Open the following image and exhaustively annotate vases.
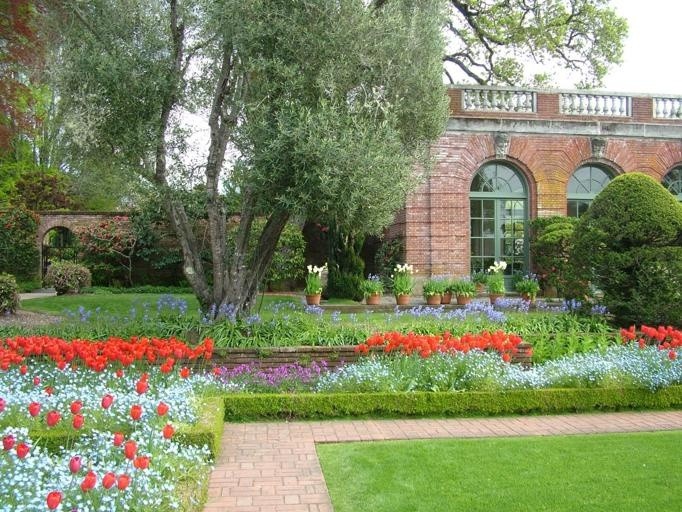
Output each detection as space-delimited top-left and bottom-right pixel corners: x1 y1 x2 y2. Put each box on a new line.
489 293 504 304
305 294 321 306
365 293 381 305
395 294 411 305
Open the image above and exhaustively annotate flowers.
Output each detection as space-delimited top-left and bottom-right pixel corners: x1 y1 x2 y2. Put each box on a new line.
303 264 323 296
389 263 420 299
487 261 508 293
361 272 385 298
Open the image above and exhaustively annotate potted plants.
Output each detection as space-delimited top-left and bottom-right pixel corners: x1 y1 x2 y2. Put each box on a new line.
517 279 540 301
422 271 489 305
